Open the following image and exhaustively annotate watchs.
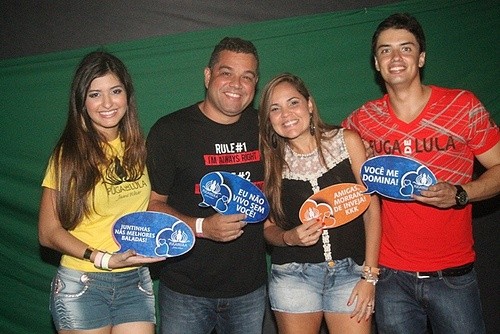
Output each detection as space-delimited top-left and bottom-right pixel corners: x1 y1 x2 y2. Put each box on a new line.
453 184 469 209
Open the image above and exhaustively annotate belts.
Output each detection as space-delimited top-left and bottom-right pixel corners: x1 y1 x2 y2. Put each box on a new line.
407 261 475 279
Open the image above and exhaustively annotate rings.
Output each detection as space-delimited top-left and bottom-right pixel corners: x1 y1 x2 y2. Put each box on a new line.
367 305 372 307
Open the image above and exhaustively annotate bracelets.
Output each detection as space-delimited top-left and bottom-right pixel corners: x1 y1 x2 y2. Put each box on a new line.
195 217 204 238
360 265 380 286
101 252 112 271
83 247 98 263
282 230 291 246
94 251 105 269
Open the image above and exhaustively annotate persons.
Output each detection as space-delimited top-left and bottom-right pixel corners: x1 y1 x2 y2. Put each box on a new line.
144 35 269 334
38 50 166 334
257 70 383 334
341 12 500 334
